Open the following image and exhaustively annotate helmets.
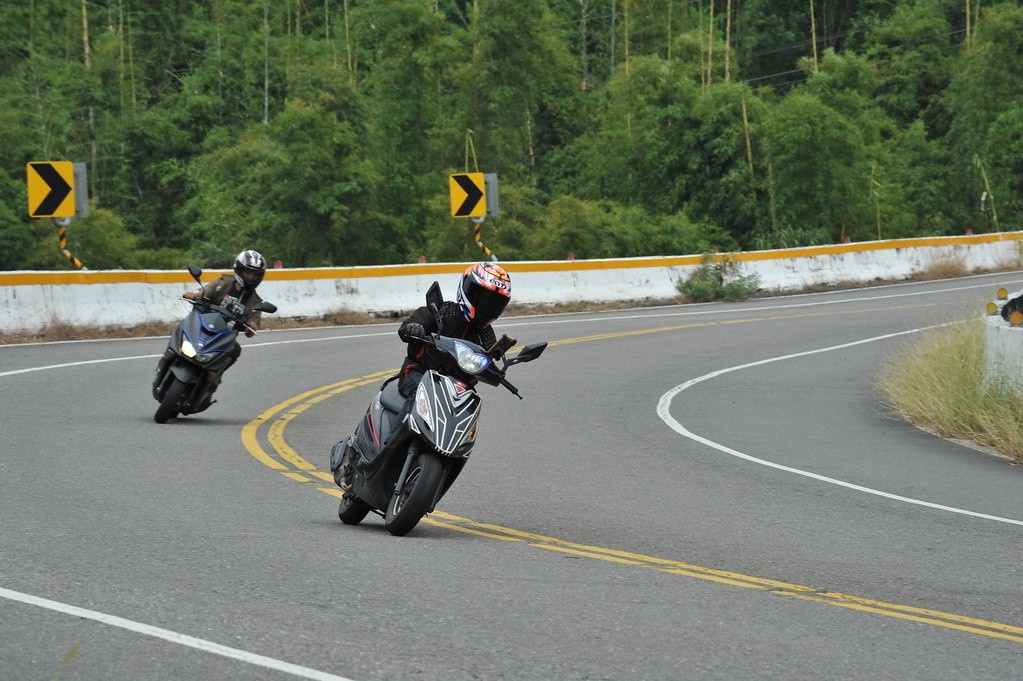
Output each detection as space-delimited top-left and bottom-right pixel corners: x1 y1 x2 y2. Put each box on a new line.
234 249 267 290
457 262 512 329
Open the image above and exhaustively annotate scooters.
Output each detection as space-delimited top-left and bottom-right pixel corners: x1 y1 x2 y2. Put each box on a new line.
151 264 278 424
328 279 549 537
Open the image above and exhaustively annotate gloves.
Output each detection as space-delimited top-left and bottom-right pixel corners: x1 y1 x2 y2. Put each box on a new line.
245 321 257 338
404 322 426 341
184 291 201 300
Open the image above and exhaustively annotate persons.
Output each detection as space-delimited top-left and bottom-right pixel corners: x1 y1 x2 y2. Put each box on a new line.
156 250 265 394
384 261 510 462
